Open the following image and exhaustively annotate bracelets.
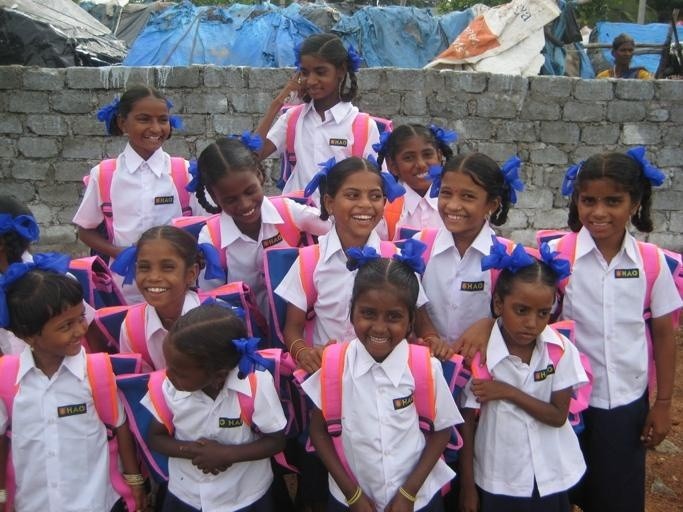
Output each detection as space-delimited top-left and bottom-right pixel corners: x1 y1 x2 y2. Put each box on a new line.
288 337 305 356
122 472 146 486
422 332 441 340
655 397 673 402
294 345 313 365
348 487 361 505
398 486 416 502
342 484 359 504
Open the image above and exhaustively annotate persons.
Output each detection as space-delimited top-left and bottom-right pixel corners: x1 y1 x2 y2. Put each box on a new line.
410 152 518 511
536 148 682 511
0 191 94 355
456 230 591 512
595 34 653 79
0 266 150 512
185 133 333 334
371 122 455 239
295 236 465 510
68 85 219 305
137 297 289 511
275 154 455 378
247 33 389 204
109 222 239 374
